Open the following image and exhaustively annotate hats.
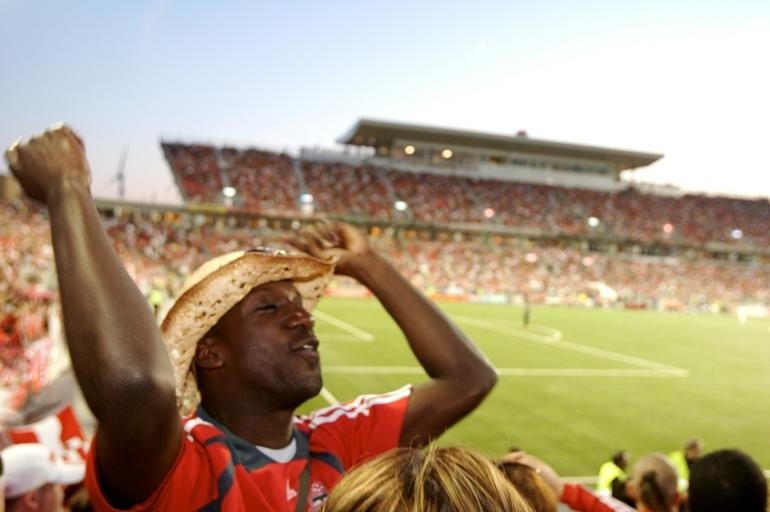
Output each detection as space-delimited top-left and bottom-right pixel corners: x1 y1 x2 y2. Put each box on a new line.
157 246 347 415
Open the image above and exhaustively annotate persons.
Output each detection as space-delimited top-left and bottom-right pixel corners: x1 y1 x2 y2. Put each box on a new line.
0 182 162 512
8 126 499 512
319 438 769 512
163 143 769 317
